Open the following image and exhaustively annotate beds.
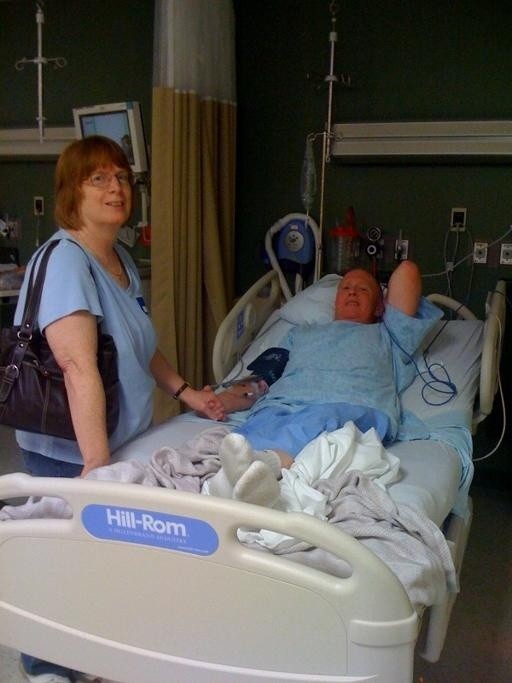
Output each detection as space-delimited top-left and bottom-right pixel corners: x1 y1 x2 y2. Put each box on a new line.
1 275 507 683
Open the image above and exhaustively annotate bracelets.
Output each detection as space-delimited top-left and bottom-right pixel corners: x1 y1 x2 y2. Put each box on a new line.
173 381 193 400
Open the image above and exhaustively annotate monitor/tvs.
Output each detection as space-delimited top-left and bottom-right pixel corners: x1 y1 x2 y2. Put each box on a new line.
72 101 149 173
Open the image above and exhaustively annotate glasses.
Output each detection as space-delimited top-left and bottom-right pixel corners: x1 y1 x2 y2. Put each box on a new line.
81 170 135 186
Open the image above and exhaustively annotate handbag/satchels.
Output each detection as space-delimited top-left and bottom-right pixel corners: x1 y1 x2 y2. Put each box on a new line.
0 325 119 441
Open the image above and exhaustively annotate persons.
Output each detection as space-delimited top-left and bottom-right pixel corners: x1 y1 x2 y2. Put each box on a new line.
199 259 446 532
13 135 226 683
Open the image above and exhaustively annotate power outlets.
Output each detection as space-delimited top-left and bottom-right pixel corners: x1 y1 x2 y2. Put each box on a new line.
450 208 466 232
394 238 408 261
